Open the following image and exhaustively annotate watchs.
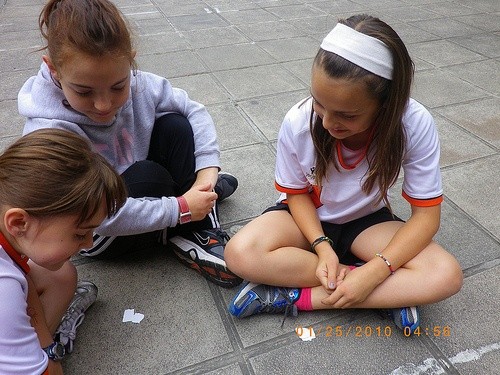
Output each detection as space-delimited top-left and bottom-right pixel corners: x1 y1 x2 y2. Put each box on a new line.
175 196 192 224
42 342 66 361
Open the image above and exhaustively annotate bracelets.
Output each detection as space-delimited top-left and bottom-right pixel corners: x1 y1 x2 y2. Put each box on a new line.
312 236 334 249
376 253 394 274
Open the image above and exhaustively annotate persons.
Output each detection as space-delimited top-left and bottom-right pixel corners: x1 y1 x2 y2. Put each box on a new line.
0 127 121 375
224 15 463 335
18 1 242 287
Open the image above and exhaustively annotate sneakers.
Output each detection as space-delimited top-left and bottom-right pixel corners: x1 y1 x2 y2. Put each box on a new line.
53 281 98 353
392 306 421 334
169 228 243 289
229 281 301 328
214 171 239 201
